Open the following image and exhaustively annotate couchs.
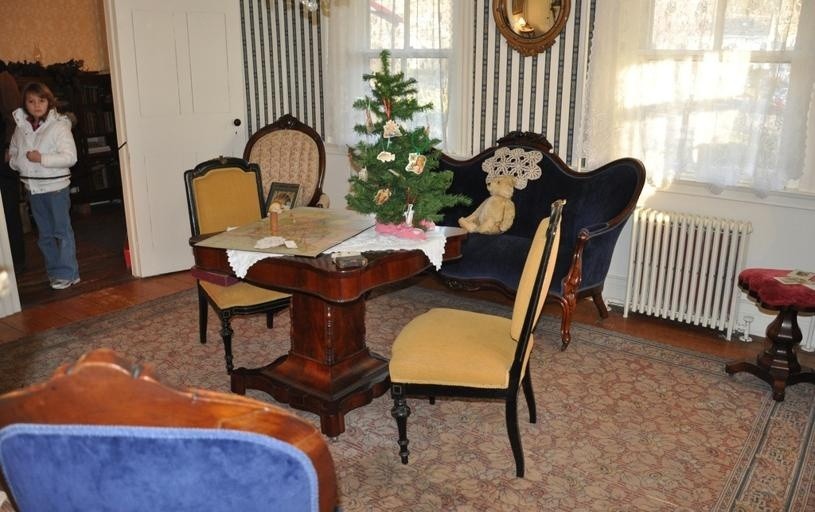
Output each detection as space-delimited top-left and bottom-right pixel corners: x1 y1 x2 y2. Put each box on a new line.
423 131 648 351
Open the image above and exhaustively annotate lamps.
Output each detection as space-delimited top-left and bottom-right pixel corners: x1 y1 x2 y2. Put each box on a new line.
0 350 340 511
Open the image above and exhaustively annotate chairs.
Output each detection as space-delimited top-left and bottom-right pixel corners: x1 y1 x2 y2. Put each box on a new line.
0 350 340 511
183 112 565 477
51 275 81 290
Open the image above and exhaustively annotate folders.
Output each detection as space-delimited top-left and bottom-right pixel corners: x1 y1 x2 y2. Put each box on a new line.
623 205 752 342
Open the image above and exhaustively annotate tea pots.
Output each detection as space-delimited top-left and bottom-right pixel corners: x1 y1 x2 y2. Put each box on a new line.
492 0 571 57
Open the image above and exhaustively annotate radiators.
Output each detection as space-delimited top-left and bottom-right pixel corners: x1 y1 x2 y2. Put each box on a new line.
623 205 752 342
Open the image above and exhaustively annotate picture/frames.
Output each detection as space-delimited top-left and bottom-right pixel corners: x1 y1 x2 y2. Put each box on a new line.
518 26 535 38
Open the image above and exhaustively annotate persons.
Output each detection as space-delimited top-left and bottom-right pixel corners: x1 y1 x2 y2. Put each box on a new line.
8 80 82 291
1 68 30 277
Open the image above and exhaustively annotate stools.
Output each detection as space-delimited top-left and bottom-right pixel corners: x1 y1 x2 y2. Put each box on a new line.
726 269 815 401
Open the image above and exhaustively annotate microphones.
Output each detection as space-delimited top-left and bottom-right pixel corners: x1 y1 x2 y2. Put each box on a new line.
726 269 815 401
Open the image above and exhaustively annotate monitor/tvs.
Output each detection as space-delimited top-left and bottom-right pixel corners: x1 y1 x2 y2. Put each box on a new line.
265 181 303 217
774 268 815 291
67 82 122 194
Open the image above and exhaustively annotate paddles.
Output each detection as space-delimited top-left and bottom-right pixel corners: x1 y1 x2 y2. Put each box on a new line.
0 285 815 512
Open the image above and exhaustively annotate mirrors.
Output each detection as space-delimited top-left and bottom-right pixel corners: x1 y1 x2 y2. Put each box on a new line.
492 0 571 57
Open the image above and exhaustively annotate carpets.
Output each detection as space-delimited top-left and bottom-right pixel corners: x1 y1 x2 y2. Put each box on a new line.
0 285 815 512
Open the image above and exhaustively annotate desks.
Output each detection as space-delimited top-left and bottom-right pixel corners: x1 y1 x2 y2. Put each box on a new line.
518 26 535 38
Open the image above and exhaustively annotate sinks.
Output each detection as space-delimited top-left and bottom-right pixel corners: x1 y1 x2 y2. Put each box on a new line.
423 131 648 351
